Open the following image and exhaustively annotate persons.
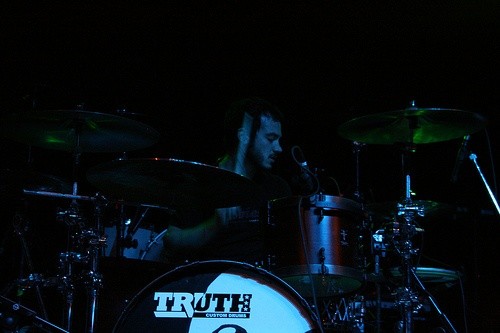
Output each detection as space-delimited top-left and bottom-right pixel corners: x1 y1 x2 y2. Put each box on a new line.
151 92 306 271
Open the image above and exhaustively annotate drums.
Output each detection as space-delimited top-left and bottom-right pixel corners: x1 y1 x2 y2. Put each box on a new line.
110 259 324 333
258 193 369 300
359 266 468 333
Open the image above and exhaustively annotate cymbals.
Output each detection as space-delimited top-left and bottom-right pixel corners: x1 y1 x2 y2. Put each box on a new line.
77 157 256 211
367 199 450 214
3 108 175 155
337 106 481 145
